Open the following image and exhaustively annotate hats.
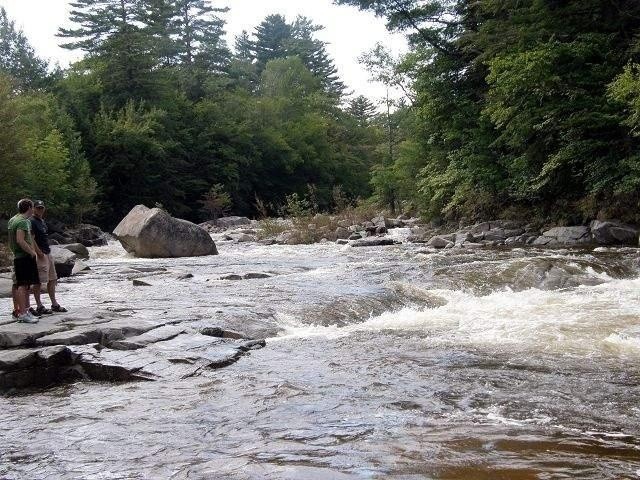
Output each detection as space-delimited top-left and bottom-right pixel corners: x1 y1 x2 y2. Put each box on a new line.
34 200 45 208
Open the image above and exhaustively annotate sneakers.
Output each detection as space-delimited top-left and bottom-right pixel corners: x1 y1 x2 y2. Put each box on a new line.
12 307 41 323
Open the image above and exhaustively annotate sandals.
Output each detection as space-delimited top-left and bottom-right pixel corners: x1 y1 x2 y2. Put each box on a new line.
37 304 53 314
51 303 68 312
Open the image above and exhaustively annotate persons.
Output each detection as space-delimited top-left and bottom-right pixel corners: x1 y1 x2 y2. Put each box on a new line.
28 199 68 314
